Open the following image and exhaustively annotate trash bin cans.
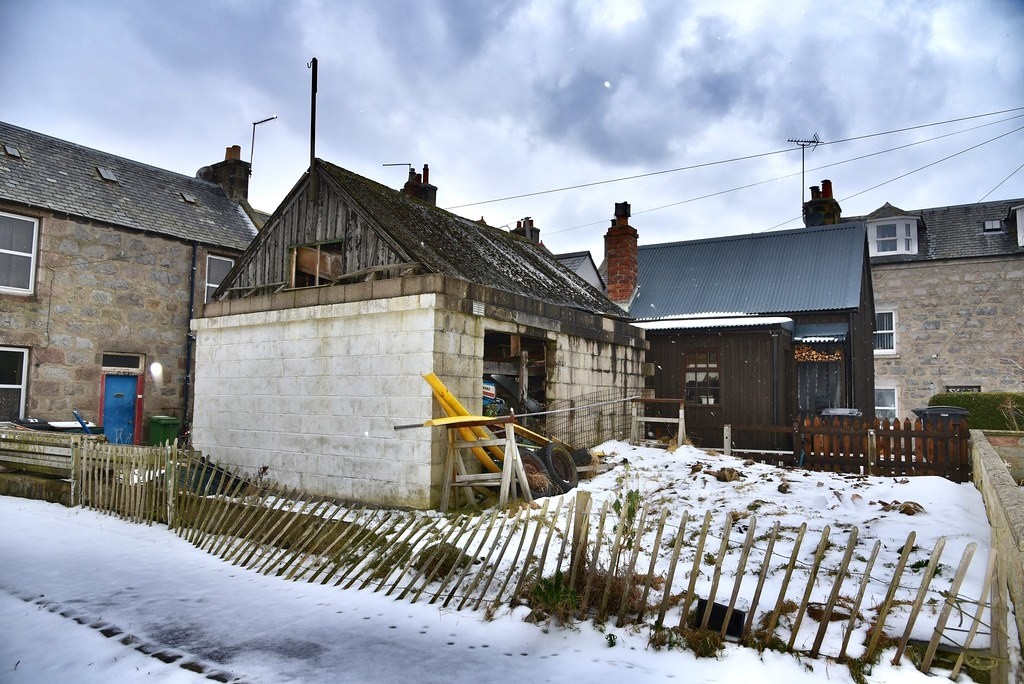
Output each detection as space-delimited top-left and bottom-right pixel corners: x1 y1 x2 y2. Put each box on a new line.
822 407 863 474
911 407 970 475
149 415 182 447
